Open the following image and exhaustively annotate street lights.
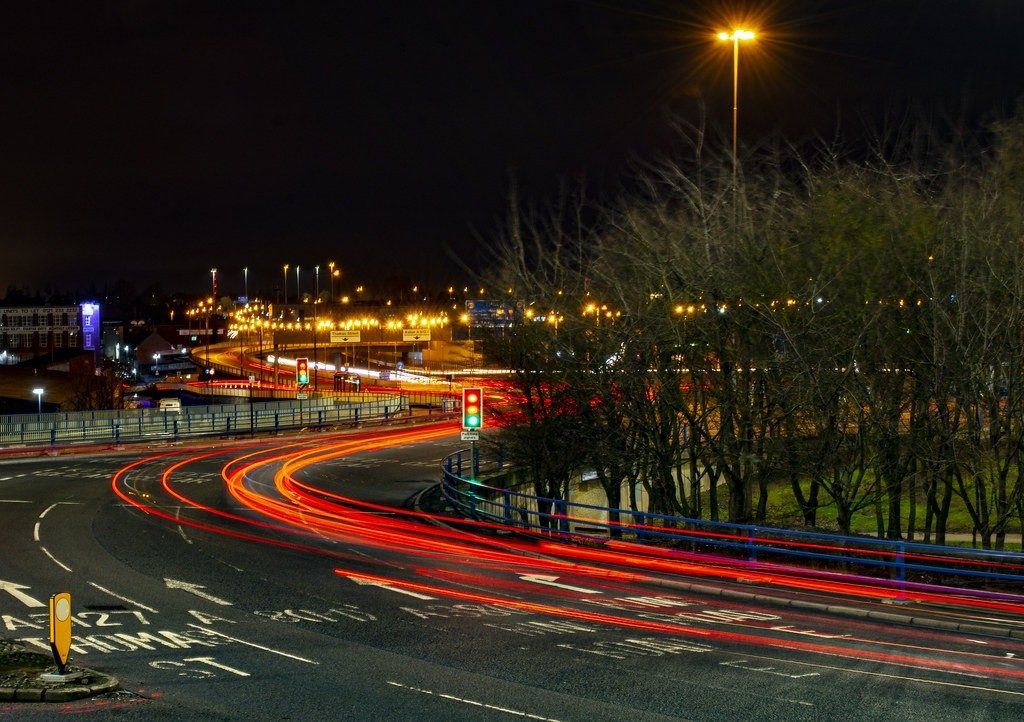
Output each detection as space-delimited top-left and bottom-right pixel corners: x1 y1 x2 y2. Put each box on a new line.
184 298 221 375
715 25 761 245
495 303 621 377
226 303 450 381
31 387 46 422
459 313 473 340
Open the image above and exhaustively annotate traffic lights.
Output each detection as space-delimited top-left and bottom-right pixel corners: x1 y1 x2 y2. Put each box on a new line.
294 356 310 386
458 383 484 434
